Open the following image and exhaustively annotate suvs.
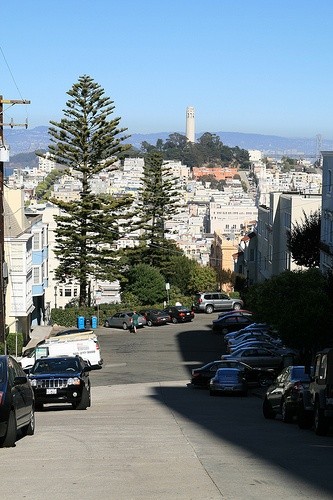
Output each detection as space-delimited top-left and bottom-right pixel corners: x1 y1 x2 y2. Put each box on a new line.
298 347 333 436
193 292 245 314
24 355 92 411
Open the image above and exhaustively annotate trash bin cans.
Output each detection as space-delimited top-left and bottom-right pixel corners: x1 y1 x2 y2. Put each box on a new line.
92 316 98 328
76 315 85 330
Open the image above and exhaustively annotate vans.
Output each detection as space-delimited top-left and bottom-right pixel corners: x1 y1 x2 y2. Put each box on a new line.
15 328 104 372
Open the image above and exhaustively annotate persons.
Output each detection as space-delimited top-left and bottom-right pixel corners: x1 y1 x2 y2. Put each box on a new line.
131 311 138 333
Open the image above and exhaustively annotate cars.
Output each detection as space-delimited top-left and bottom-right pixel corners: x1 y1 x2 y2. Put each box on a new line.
192 306 290 390
262 365 316 425
0 355 35 448
163 306 196 324
210 368 247 395
138 309 171 327
104 311 147 329
